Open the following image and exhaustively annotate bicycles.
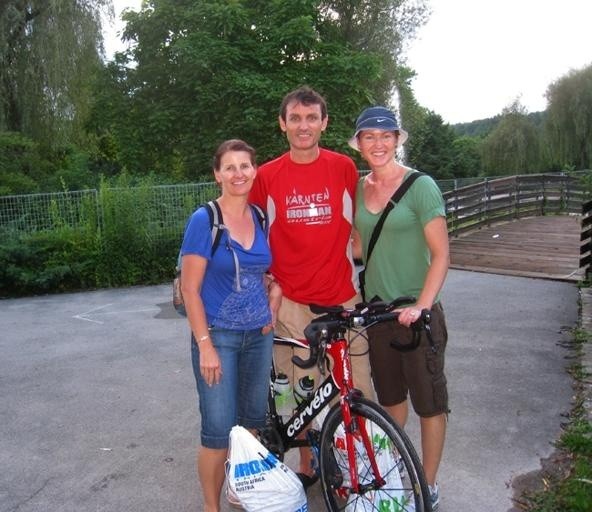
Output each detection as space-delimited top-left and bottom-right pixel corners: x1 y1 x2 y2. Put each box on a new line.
251 295 439 512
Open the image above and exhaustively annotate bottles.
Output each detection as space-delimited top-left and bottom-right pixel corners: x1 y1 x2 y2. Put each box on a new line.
274 371 294 418
285 373 316 408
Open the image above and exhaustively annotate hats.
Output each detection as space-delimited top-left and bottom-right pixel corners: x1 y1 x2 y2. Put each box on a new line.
347 106 408 153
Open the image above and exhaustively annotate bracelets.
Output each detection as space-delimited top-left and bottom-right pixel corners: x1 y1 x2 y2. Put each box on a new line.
267 279 280 295
196 335 209 345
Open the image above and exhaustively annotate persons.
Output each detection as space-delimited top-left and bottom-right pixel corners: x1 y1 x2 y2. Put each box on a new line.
179 138 286 512
346 107 453 511
248 84 376 492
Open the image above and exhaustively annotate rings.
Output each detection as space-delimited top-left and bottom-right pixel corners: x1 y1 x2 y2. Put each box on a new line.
410 311 415 316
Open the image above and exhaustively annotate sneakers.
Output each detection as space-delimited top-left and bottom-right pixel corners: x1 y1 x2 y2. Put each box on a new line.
295 471 318 493
427 481 440 511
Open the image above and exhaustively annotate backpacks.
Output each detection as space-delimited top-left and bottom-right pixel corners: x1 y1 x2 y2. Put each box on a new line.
173 200 268 318
